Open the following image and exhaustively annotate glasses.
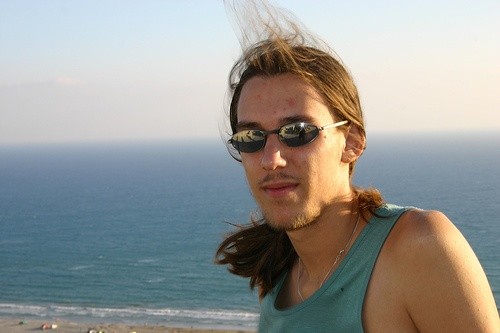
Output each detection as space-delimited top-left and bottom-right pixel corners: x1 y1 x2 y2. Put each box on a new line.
227 120 348 153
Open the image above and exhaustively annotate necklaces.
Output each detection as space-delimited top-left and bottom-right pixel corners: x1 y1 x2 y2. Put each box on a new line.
297 215 360 302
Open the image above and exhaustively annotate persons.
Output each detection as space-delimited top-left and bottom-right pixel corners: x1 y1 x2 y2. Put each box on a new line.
216 36 500 333
